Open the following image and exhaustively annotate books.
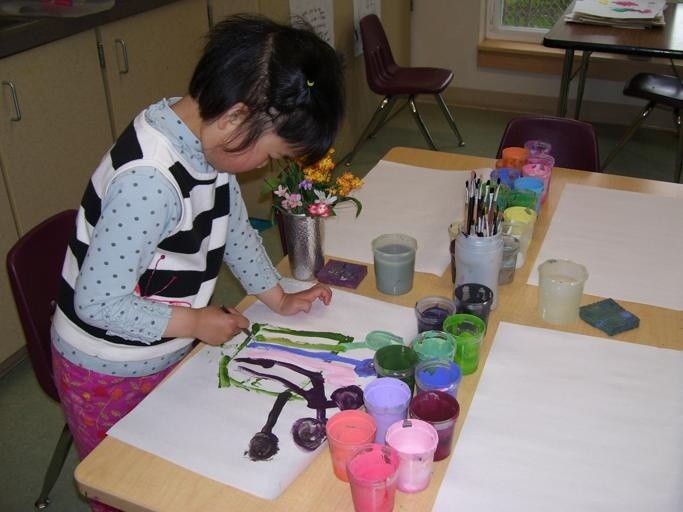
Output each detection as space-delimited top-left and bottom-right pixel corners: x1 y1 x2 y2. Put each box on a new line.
563 1 668 31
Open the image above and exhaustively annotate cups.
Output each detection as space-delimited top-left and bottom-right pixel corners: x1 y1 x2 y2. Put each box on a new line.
454 221 503 313
371 232 417 295
537 257 590 328
346 442 400 512
416 295 456 330
385 417 439 496
408 389 460 463
448 223 464 242
443 313 486 375
362 377 413 444
504 224 531 269
498 233 519 285
372 344 418 394
452 283 493 335
414 358 461 400
478 138 555 211
411 329 457 364
448 238 456 285
503 204 537 252
325 410 377 484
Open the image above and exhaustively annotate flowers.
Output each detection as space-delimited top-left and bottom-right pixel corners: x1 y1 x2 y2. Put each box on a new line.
261 155 367 218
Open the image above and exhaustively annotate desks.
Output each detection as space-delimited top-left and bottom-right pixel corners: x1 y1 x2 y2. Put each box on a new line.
541 0 683 120
73 146 683 512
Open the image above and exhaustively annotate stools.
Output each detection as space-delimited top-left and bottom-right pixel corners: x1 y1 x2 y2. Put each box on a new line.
600 70 682 183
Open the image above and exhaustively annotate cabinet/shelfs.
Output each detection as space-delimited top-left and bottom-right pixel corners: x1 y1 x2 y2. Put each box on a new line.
0 168 33 384
1 30 117 240
98 2 215 143
208 1 414 221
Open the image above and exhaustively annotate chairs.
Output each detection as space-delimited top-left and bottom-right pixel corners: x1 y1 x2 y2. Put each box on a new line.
343 13 467 167
495 116 601 172
5 208 82 512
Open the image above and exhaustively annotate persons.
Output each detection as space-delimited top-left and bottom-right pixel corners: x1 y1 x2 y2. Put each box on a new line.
50 12 349 510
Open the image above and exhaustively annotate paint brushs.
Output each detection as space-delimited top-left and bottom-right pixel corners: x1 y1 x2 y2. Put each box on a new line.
219 305 254 339
464 170 504 237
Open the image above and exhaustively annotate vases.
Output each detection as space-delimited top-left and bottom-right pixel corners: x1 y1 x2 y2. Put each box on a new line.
284 212 326 282
